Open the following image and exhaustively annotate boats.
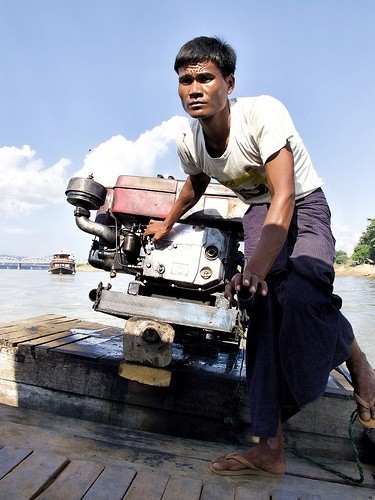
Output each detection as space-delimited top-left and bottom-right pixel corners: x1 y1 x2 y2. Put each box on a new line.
48 249 76 275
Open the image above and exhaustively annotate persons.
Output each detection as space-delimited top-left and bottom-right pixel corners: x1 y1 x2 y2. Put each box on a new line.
142 33 375 478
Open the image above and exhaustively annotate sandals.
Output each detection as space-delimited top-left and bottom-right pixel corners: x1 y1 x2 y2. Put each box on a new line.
353 389 375 429
210 450 286 478
